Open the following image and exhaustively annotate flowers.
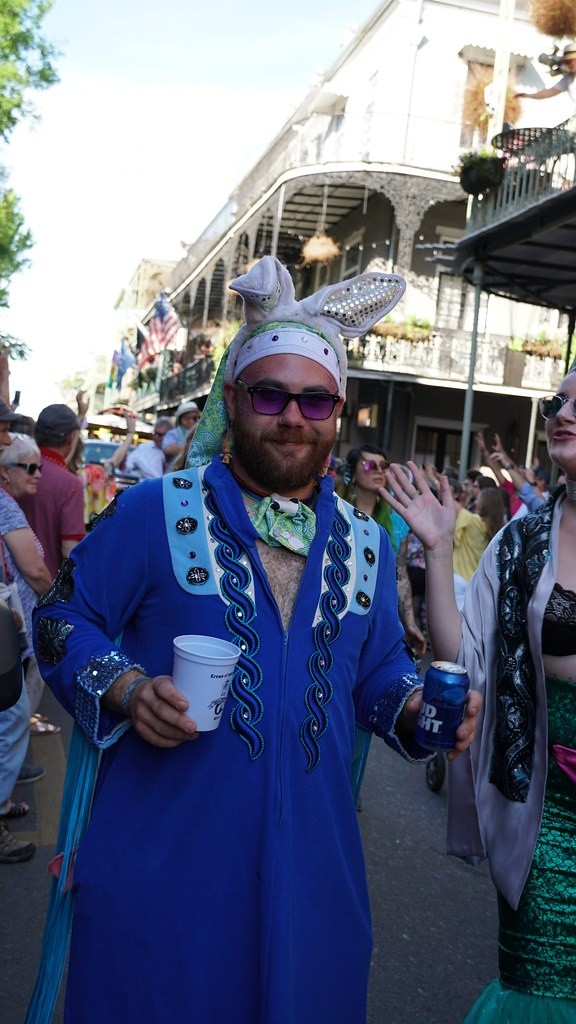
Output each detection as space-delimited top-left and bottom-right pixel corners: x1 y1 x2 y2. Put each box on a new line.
447 142 502 177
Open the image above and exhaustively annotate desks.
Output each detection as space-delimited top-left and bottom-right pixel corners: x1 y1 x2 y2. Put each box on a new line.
493 127 576 201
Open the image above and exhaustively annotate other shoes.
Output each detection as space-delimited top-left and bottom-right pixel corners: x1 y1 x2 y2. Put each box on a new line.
0 822 36 863
30 713 62 736
15 764 45 784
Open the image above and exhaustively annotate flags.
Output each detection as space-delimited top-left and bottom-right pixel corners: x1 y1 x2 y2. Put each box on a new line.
138 293 182 367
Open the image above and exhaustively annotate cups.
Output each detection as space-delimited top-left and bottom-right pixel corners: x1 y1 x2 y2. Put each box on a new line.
170 635 242 732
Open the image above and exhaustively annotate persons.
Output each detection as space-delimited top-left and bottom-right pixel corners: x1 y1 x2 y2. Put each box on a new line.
335 431 566 660
0 390 136 862
380 367 576 1024
125 417 174 483
515 43 576 103
161 401 201 474
25 254 483 1024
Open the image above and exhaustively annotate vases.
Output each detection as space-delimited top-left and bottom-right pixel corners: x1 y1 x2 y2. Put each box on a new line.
460 156 506 194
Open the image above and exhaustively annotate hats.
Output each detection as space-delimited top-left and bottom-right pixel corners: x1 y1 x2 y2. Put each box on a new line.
173 401 198 420
0 397 22 421
36 403 81 435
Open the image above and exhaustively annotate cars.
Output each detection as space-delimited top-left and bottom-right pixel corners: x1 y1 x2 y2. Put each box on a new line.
79 438 138 495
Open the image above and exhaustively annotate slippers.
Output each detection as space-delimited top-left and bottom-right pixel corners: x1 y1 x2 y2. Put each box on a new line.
0 799 31 818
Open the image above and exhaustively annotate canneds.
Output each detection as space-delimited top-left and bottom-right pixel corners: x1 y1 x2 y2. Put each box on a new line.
414 660 470 753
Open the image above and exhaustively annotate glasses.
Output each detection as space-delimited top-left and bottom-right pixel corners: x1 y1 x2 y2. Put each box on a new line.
354 460 390 472
236 378 341 419
538 395 576 420
9 462 43 476
155 431 165 436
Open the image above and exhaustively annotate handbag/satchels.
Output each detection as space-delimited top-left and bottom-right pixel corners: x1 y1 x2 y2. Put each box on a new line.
0 597 27 713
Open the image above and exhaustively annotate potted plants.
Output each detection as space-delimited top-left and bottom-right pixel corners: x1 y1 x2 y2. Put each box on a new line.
372 311 438 342
506 329 563 359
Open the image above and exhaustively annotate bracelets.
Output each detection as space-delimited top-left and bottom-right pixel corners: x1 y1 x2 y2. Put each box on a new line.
506 464 514 471
121 677 151 713
